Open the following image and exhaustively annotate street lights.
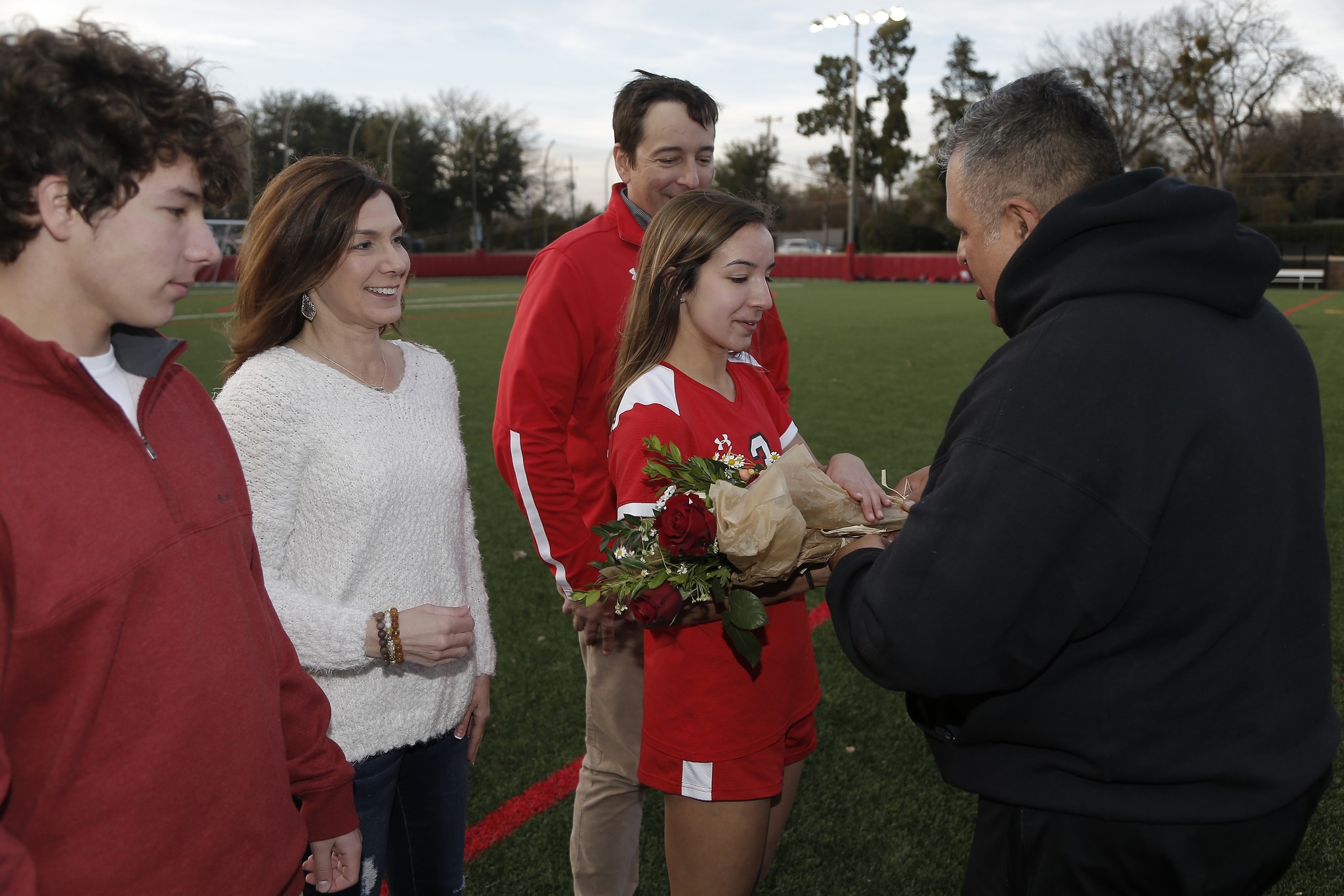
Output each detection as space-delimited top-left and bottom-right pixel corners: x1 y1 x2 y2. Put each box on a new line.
811 6 908 283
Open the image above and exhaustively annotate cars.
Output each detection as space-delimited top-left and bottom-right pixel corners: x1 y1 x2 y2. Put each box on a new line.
204 219 247 256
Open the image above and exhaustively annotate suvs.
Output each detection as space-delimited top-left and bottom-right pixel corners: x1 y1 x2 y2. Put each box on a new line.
777 238 840 255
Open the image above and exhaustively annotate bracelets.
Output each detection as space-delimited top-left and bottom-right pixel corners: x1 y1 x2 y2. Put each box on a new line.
372 607 404 670
804 566 815 592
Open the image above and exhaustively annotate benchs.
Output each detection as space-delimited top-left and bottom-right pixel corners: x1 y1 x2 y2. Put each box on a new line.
1269 267 1324 291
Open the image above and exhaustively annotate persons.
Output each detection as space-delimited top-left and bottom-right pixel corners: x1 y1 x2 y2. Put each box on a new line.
212 154 500 895
823 67 1336 895
0 15 370 894
493 67 795 896
608 188 893 896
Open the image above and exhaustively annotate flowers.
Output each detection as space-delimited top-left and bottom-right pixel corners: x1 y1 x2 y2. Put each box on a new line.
559 437 910 671
